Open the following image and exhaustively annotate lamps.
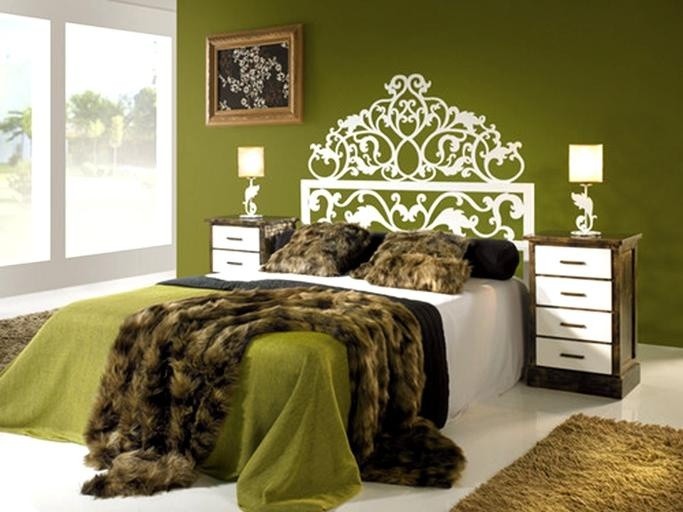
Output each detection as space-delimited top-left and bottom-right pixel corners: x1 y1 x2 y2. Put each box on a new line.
567 142 603 236
236 146 264 218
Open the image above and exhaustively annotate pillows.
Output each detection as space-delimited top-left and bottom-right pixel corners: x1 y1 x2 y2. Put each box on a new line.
262 222 520 295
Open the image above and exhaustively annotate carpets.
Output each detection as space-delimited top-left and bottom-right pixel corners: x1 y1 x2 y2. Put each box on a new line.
0 308 55 374
448 411 683 512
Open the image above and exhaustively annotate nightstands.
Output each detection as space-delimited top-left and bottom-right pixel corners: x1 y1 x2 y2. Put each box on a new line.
204 215 300 273
522 232 642 399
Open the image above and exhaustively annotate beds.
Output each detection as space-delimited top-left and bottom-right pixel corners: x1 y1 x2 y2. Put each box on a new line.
0 71 536 512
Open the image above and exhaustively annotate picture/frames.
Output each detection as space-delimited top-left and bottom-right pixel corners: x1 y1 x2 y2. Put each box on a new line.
204 22 305 127
